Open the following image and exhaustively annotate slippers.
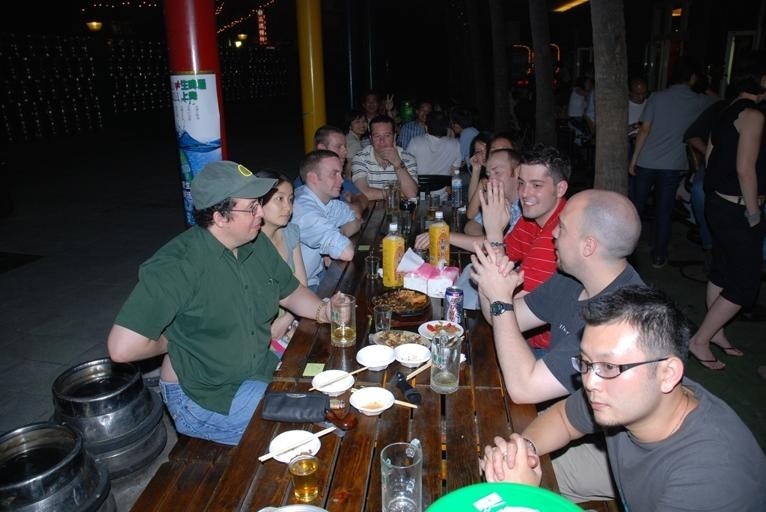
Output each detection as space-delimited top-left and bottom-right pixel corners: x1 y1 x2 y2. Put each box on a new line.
690 353 725 368
712 345 746 357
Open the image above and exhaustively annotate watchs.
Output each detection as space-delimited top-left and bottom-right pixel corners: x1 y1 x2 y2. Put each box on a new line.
488 301 514 316
386 109 395 112
520 436 539 456
395 160 408 171
634 122 636 132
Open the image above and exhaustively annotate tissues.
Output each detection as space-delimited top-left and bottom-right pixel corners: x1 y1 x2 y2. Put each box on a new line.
397 247 438 294
428 267 459 298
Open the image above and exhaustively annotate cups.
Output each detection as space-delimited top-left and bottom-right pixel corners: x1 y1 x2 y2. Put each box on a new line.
379 439 425 512
427 193 441 220
429 336 463 395
382 181 400 216
374 304 393 332
288 453 321 503
331 294 357 348
365 255 381 280
451 209 463 233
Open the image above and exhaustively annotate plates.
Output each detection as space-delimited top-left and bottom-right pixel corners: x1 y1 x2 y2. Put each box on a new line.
269 429 323 464
367 286 433 317
373 330 432 350
418 319 466 341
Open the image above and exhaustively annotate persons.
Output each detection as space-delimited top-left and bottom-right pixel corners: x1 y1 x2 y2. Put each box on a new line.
468 135 492 203
341 112 363 178
478 140 571 360
478 285 766 512
106 160 356 447
292 123 369 234
404 108 461 183
465 135 513 220
433 94 458 119
363 90 396 126
288 149 362 293
585 88 595 171
463 147 526 239
681 70 765 323
451 112 481 176
568 76 592 136
628 35 719 270
629 73 650 200
687 50 765 378
467 188 655 504
351 116 419 200
396 97 432 150
254 170 308 352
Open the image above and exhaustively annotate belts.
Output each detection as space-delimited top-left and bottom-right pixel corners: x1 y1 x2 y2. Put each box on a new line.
713 187 764 210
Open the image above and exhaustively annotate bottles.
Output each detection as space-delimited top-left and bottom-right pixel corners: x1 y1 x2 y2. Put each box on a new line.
452 167 464 210
383 225 405 289
444 288 465 324
431 213 450 271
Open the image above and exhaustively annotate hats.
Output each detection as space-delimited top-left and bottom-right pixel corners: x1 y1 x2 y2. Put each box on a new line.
190 159 281 208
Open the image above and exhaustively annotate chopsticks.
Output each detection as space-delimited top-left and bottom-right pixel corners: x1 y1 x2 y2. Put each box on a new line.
309 365 369 394
351 385 419 410
257 426 337 463
406 362 433 382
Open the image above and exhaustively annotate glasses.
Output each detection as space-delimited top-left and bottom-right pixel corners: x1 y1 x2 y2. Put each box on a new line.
572 354 677 378
220 198 269 212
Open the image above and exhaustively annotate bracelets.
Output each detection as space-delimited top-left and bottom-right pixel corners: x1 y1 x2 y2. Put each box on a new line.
746 209 762 222
315 298 330 322
488 242 505 247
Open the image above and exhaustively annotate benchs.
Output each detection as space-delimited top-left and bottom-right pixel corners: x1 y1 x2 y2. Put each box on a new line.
129 435 237 512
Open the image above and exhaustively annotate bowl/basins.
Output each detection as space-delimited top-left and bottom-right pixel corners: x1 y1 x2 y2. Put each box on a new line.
348 386 397 417
312 369 356 398
394 343 432 369
357 344 396 372
